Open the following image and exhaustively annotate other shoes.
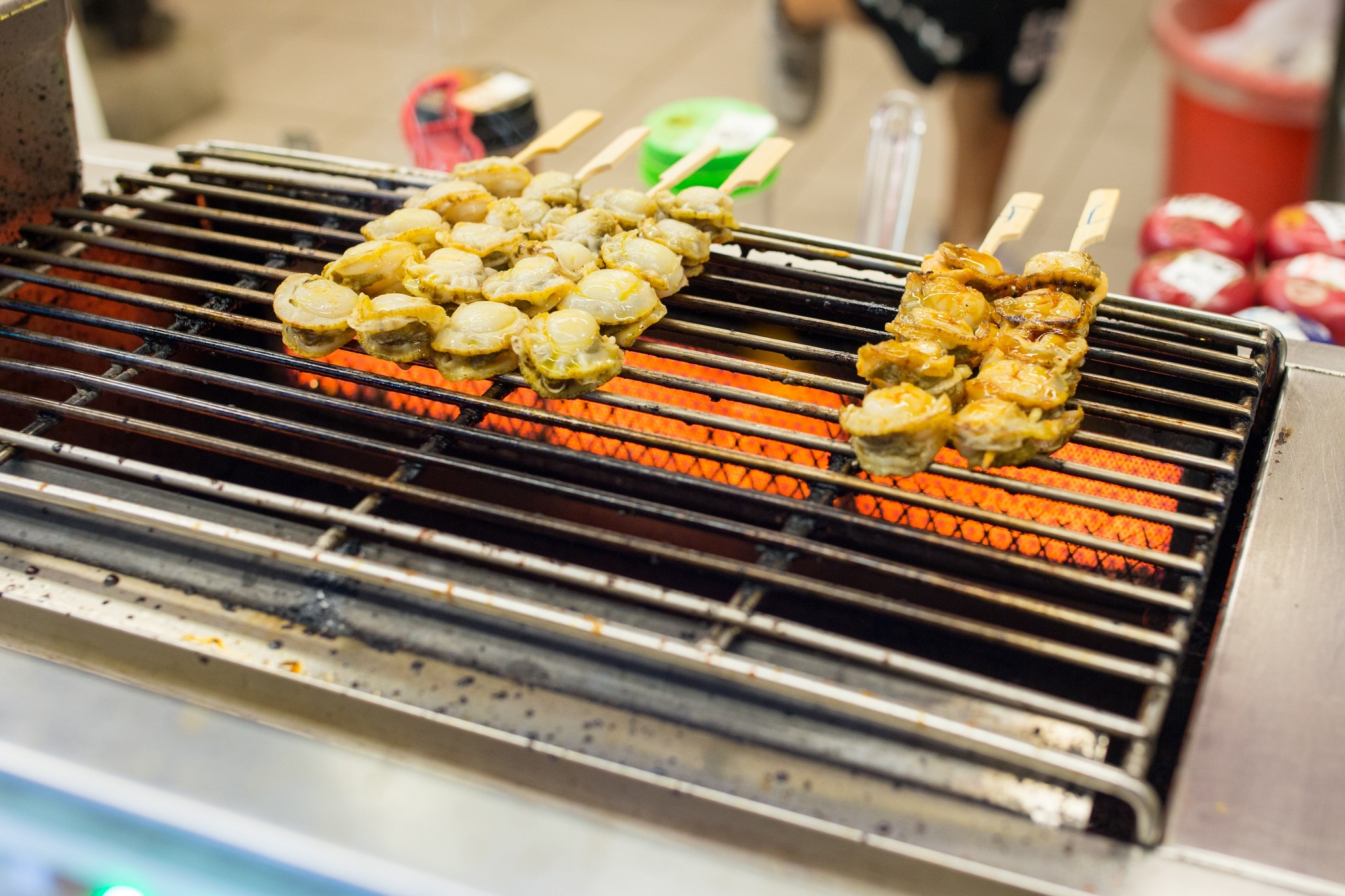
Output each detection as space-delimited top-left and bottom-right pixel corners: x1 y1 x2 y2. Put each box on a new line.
771 0 826 127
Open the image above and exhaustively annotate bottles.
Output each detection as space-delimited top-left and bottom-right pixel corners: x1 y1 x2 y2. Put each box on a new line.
1131 190 1344 347
640 101 784 230
410 67 540 157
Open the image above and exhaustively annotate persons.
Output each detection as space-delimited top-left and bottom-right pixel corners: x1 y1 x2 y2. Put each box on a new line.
755 0 1072 251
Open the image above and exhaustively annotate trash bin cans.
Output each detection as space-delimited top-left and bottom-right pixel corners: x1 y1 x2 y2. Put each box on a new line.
1152 1 1345 222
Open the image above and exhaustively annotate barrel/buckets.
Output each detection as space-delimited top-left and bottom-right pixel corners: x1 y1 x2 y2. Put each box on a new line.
1157 2 1335 231
